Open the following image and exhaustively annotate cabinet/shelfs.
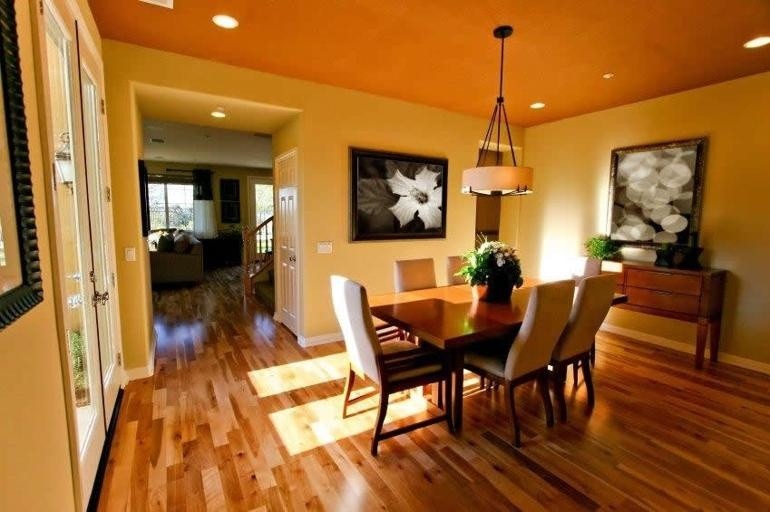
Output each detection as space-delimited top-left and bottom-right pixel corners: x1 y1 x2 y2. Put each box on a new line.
600 259 727 371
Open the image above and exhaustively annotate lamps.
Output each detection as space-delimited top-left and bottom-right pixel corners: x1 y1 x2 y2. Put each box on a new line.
459 25 535 197
54 131 74 195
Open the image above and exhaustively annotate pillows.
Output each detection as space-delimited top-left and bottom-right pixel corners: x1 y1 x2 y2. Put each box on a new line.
156 229 189 252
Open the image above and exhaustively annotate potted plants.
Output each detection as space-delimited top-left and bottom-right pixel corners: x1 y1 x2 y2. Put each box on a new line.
583 232 627 276
67 330 90 408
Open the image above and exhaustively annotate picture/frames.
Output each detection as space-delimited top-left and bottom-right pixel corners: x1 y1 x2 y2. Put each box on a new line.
348 147 449 244
602 135 707 268
0 0 44 334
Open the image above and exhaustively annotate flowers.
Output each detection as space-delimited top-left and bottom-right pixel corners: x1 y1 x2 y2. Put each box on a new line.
357 161 444 232
454 234 524 290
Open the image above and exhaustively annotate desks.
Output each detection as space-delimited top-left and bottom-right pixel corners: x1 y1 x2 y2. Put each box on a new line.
363 275 624 440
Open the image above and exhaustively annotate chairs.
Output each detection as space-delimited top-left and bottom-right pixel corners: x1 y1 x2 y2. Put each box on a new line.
444 252 484 289
386 254 436 298
327 271 458 455
452 275 558 446
550 274 620 426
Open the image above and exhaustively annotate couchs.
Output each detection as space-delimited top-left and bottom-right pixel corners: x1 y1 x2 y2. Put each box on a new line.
148 226 204 286
